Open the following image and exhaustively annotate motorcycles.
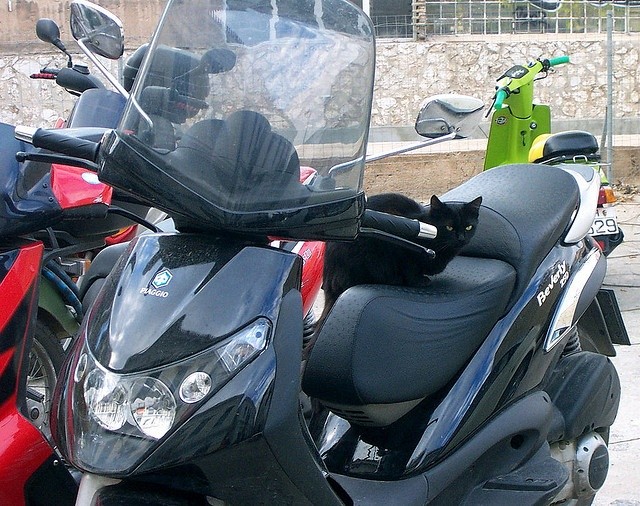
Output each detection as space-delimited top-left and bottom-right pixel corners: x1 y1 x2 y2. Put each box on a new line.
0 123 152 447
1 20 328 505
486 56 624 256
15 0 621 506
